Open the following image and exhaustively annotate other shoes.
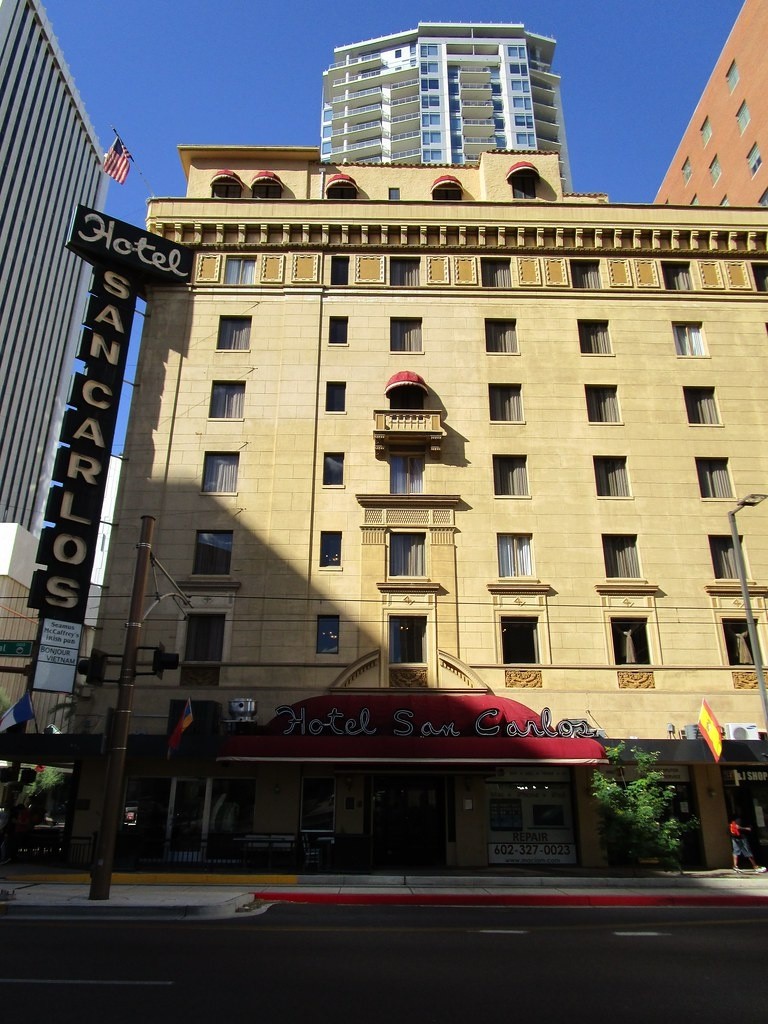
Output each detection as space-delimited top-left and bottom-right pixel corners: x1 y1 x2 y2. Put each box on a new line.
754 866 767 874
733 866 743 873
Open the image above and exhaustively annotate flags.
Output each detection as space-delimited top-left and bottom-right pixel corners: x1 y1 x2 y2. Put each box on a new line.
104 136 131 186
0 692 36 733
696 699 725 764
168 698 194 751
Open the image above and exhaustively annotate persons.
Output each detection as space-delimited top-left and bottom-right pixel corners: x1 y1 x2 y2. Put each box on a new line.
729 806 767 873
0 768 37 865
